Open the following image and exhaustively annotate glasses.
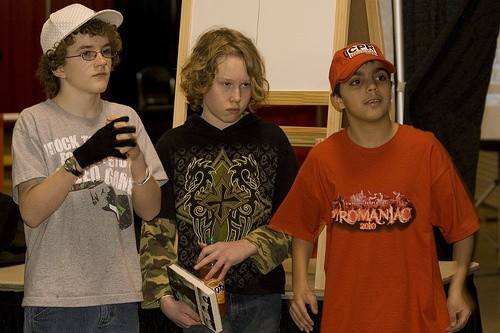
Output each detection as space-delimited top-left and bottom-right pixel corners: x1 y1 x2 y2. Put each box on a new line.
62 48 118 61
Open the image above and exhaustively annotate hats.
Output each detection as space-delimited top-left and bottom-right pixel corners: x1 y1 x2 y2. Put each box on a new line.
327 42 396 91
40 2 124 56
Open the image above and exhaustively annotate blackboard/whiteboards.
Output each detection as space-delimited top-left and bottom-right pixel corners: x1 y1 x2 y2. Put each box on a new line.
172 0 352 105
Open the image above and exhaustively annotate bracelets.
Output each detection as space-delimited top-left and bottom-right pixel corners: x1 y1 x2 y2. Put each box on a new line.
157 295 174 303
132 167 152 186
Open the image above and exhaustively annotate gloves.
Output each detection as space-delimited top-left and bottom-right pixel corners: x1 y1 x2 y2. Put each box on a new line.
72 115 136 170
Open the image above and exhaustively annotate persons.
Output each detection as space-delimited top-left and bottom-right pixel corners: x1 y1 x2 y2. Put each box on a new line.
11 3 168 333
139 26 301 333
200 295 215 330
269 43 480 333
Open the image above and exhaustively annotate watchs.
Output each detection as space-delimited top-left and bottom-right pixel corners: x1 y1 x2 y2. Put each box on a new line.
64 157 83 178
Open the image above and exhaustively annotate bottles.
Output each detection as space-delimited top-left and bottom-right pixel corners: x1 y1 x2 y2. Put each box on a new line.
199 237 226 319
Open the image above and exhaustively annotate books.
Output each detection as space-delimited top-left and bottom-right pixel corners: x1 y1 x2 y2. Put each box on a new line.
167 262 223 333
